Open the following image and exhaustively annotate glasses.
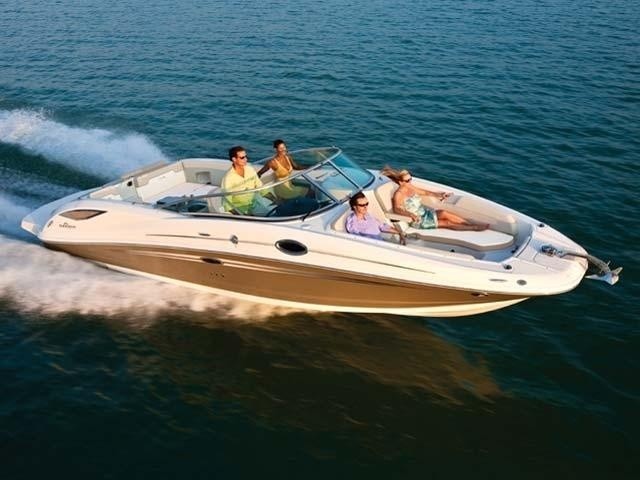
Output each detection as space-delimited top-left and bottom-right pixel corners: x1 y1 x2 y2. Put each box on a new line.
359 203 368 206
239 155 247 159
402 178 411 182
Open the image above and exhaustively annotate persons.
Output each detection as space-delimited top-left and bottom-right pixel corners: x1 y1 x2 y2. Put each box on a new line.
381 165 490 231
221 146 280 217
346 192 405 245
257 139 315 200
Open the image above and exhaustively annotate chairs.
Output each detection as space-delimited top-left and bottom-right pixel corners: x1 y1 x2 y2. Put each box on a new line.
375 181 516 254
205 187 234 215
333 191 475 260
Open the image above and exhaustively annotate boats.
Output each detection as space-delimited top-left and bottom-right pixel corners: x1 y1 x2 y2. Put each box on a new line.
15 145 622 321
